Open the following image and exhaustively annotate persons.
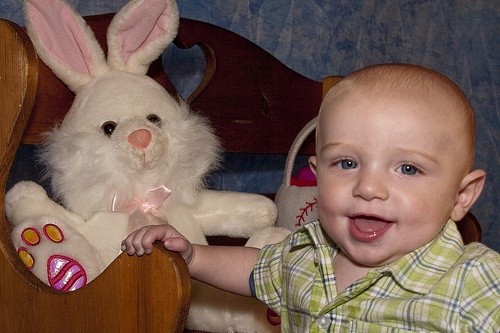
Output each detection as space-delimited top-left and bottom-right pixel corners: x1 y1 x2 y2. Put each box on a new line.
121 63 499 333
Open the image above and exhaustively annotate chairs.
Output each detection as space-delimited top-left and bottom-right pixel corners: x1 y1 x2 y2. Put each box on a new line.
0 13 482 333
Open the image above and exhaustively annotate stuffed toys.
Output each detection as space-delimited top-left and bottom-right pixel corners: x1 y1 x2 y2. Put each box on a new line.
4 0 293 333
243 116 320 251
